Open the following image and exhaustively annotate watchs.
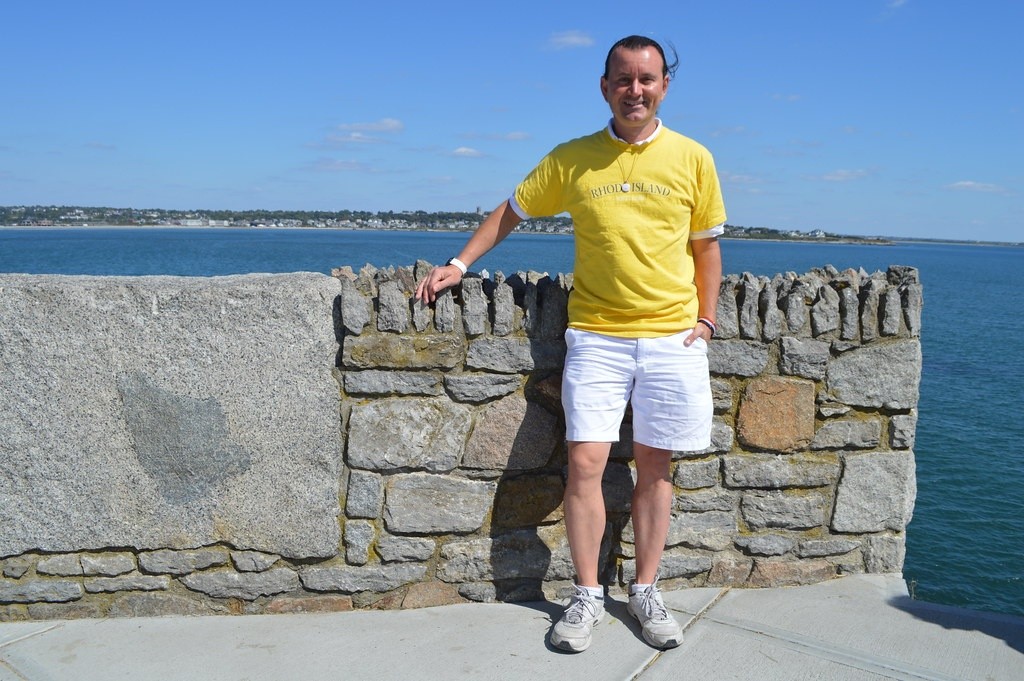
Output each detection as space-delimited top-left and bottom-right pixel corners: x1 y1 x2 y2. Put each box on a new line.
446 257 467 277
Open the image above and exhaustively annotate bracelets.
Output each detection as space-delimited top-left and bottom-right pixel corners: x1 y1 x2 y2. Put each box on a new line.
697 317 717 336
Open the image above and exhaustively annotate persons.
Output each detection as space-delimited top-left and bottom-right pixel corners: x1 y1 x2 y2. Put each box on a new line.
415 35 727 653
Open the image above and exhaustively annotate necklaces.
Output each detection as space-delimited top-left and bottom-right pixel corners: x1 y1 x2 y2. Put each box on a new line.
617 129 653 191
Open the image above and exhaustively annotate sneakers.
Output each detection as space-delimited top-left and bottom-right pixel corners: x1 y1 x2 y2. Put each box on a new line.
626 573 684 649
550 582 604 652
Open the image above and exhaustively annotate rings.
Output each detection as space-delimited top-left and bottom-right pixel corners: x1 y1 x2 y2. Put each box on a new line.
420 277 427 281
